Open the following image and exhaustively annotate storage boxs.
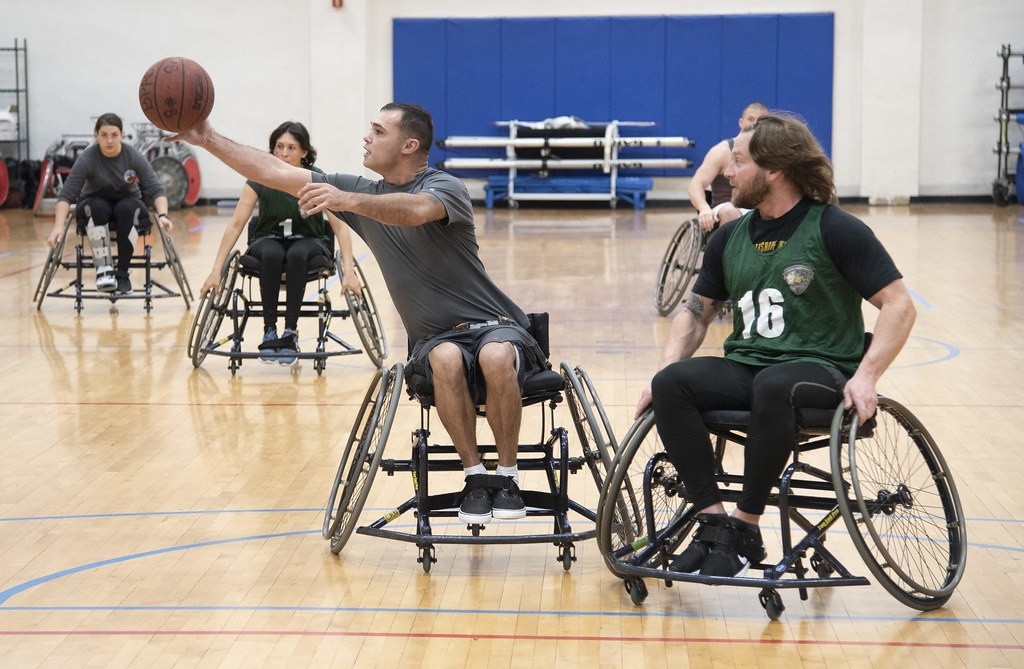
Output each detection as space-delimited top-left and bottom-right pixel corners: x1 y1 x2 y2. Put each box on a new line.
0 105 19 141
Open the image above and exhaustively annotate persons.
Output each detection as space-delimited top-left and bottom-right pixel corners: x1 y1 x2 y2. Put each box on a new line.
198 122 362 368
633 113 917 582
46 113 172 295
165 104 547 522
688 103 768 232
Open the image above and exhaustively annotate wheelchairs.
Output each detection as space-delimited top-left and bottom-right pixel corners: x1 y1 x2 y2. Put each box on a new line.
652 216 736 326
137 122 202 208
27 135 94 216
31 203 198 309
185 251 389 377
322 311 644 575
592 339 970 624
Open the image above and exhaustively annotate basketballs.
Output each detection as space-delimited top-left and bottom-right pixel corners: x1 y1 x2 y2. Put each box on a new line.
138 57 215 133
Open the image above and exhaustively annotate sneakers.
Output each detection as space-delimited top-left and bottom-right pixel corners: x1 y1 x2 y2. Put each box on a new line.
492 484 526 518
698 524 769 578
277 329 299 366
113 270 132 295
257 325 279 362
666 512 731 575
458 487 492 523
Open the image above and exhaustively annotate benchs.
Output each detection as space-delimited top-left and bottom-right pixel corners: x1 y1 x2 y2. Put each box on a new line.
484 175 654 211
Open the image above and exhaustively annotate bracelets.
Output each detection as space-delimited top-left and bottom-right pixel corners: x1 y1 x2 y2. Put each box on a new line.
159 214 165 217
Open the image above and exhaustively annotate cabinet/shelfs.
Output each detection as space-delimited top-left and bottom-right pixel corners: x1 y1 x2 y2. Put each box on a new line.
991 44 1024 208
0 38 30 212
